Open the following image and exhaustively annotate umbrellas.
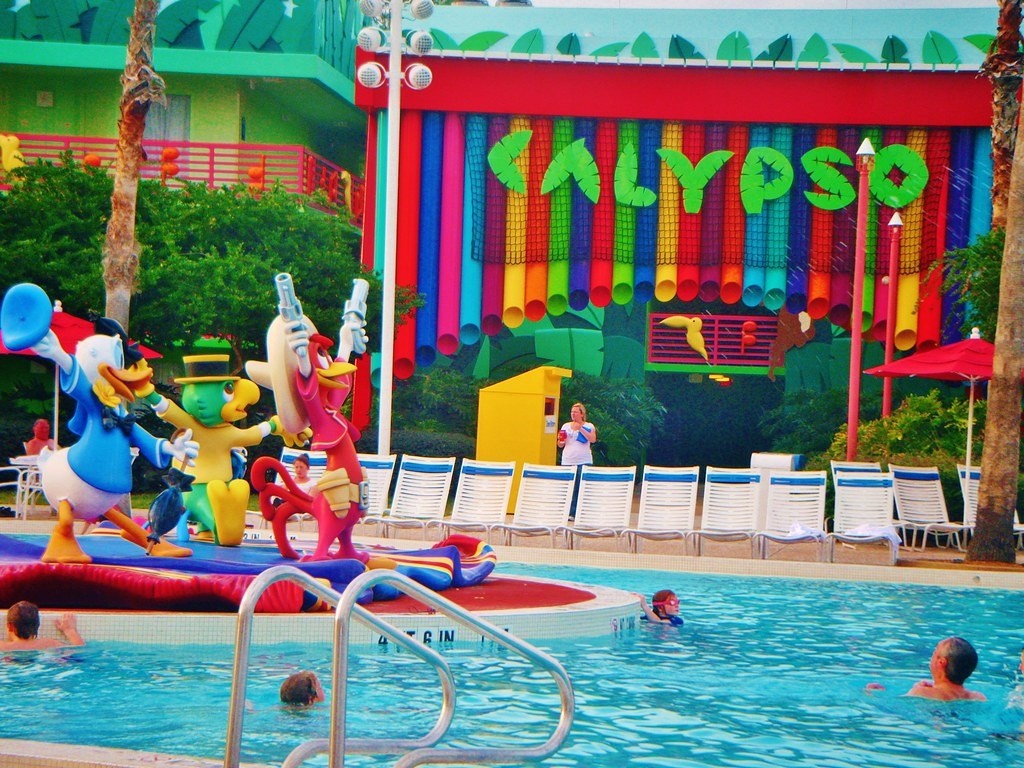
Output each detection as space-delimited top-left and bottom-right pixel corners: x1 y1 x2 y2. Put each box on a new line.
861 327 1024 549
0 299 162 454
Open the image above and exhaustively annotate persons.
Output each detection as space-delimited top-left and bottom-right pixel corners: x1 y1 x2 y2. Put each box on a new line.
271 455 320 509
555 402 597 521
281 671 326 709
632 590 684 639
0 601 85 652
867 637 986 701
25 419 61 453
1008 647 1024 707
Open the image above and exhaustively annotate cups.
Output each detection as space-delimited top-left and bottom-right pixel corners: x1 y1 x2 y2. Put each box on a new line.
559 430 565 443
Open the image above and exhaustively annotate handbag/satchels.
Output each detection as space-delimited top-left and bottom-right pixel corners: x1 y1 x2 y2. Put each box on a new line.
576 426 591 444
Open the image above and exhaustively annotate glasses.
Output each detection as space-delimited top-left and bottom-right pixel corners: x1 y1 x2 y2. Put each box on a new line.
652 599 681 607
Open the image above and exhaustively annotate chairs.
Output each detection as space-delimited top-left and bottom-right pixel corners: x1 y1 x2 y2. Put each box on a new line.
888 464 1024 552
231 447 902 567
0 447 140 521
